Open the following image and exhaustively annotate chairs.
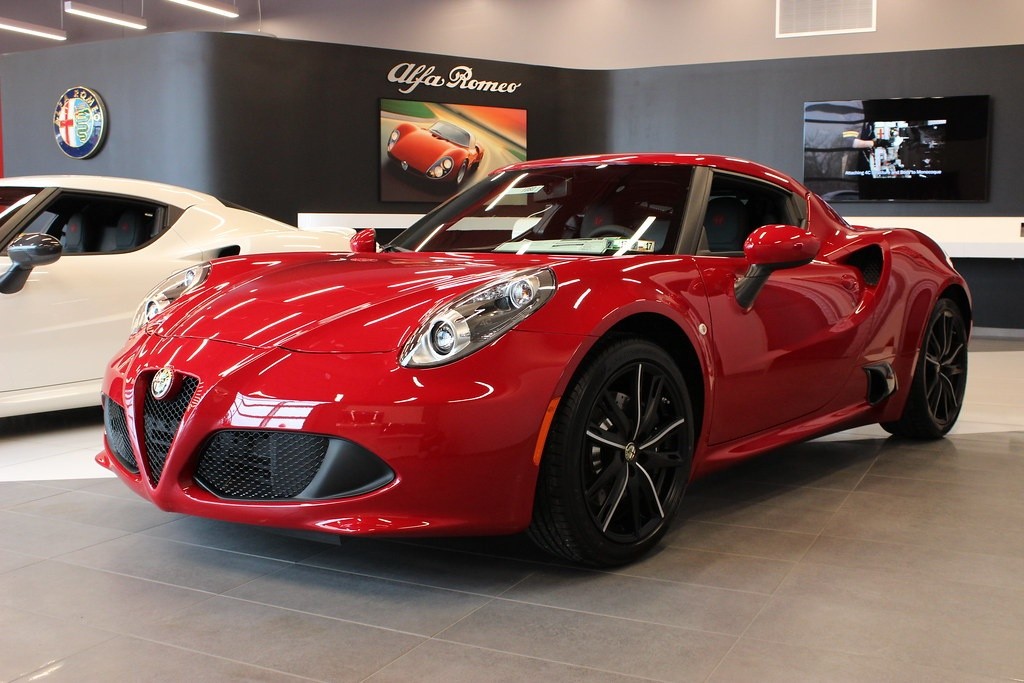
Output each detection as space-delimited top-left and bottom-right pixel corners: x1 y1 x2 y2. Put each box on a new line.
110 210 147 252
61 211 91 255
579 192 622 241
703 197 746 253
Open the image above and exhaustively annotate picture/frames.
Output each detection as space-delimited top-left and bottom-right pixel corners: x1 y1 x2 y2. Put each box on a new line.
377 97 531 207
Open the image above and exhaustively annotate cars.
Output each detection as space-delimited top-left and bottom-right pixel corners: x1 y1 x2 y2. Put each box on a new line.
0 174 382 422
386 118 485 188
92 152 973 568
